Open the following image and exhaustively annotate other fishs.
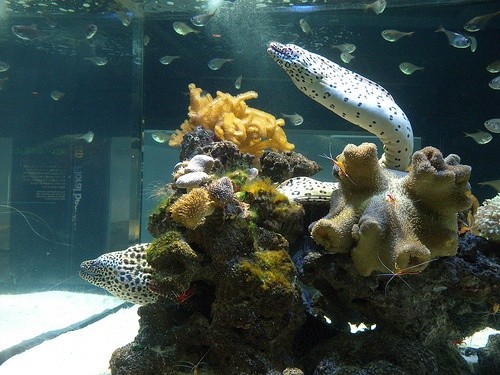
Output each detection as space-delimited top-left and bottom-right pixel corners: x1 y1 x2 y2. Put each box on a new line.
78 242 159 305
1 0 500 144
268 42 414 208
